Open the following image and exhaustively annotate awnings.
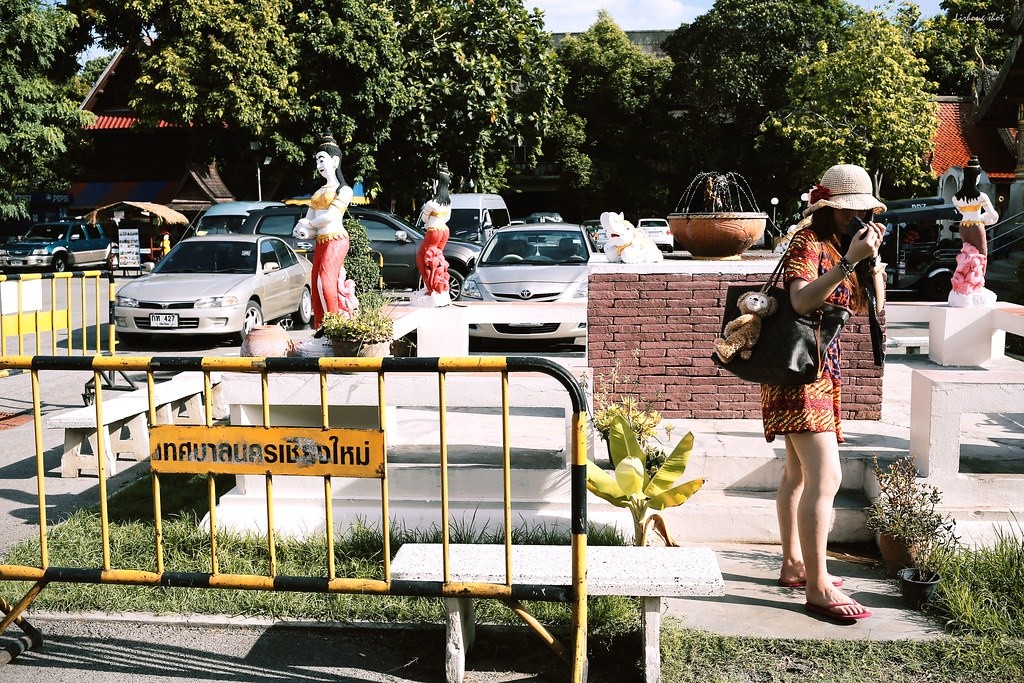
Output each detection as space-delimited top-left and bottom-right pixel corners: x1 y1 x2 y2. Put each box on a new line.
82 202 189 259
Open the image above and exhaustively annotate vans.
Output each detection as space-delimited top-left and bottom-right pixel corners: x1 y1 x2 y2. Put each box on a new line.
414 193 512 248
190 201 295 235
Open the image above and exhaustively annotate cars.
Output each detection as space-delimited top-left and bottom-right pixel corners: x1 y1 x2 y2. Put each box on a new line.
456 223 605 347
581 220 608 253
112 232 315 345
240 205 482 302
635 218 676 254
529 212 564 224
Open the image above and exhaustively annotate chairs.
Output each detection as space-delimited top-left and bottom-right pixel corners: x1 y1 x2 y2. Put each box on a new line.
501 241 531 258
555 238 572 257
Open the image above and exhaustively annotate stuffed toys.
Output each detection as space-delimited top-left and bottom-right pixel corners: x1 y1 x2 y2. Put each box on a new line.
714 291 777 364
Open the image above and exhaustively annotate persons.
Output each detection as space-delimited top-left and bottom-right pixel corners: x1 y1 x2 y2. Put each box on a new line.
295 130 353 331
760 164 886 619
952 155 998 257
416 161 452 295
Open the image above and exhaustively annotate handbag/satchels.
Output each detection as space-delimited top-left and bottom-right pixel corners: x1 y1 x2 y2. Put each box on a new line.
710 229 854 387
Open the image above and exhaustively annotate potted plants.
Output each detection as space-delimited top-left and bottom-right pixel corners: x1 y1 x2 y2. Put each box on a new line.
321 312 394 358
863 451 961 608
579 347 676 470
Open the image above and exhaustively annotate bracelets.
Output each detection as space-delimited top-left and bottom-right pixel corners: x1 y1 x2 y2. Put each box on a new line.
838 256 855 277
309 222 318 228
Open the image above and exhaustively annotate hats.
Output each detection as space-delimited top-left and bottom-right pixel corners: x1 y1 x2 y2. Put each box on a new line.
803 164 887 218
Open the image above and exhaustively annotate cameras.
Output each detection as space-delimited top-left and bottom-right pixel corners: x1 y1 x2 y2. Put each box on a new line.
847 216 868 240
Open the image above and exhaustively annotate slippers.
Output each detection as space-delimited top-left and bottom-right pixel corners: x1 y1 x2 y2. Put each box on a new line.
804 598 872 619
779 572 843 587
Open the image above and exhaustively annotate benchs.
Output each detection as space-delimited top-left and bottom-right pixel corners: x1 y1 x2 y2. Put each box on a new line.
388 540 726 683
885 302 1024 367
47 371 231 477
909 368 1024 478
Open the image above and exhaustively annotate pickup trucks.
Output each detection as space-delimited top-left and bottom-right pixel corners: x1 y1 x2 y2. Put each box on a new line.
1 220 116 272
